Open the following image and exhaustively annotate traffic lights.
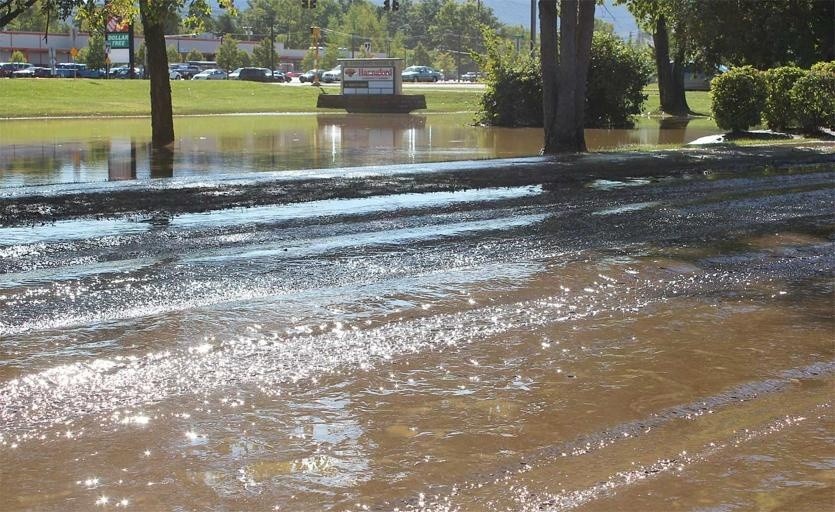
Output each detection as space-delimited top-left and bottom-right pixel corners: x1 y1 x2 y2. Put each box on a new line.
384 0 399 11
302 0 316 9
311 27 315 37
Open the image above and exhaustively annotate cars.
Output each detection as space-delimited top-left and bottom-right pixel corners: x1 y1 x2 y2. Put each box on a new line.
0 61 291 82
300 65 341 83
401 65 481 82
685 61 729 91
